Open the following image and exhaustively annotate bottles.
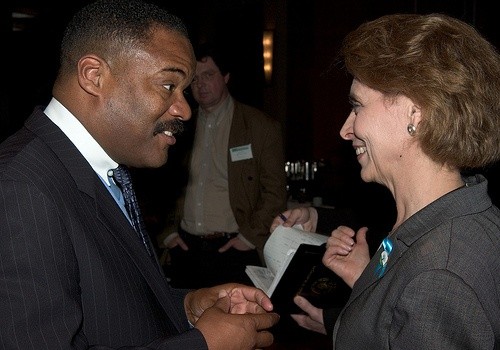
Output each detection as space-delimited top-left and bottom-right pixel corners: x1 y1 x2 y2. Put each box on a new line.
284 159 325 181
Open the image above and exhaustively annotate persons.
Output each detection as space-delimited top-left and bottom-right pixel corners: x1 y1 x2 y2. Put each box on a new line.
0 0 282 350
269 181 399 349
146 40 289 292
322 12 500 350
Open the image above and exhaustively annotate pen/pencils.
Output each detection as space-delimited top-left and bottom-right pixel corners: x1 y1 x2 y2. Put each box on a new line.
277 210 287 226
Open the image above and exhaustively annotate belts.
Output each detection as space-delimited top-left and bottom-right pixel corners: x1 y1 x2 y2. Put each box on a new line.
178 229 240 241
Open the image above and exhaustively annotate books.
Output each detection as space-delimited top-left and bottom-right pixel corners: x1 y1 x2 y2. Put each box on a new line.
244 222 352 316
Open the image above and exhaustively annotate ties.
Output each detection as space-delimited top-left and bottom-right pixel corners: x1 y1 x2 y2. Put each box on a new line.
110 166 164 284
